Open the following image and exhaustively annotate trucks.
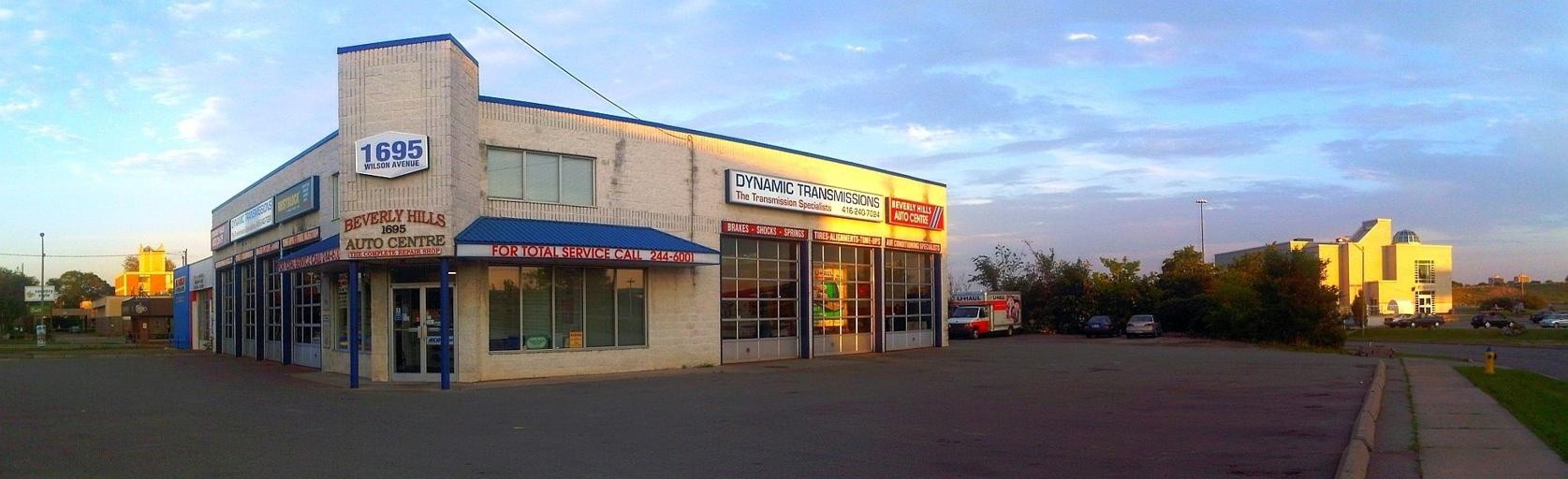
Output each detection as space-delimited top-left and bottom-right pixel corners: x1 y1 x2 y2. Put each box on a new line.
949 289 1024 339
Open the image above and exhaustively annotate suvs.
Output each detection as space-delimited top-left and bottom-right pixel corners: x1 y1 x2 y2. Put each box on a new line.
1470 310 1517 330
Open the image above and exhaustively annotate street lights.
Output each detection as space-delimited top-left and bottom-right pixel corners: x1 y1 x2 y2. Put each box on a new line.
625 275 636 318
1335 236 1367 342
39 232 46 324
1195 197 1210 255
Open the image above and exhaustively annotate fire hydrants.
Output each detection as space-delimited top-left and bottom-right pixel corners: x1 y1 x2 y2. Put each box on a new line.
1481 345 1499 375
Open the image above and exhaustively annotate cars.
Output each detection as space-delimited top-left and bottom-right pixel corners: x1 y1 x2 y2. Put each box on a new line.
1384 313 1414 328
1398 312 1447 329
1125 314 1165 338
1529 310 1555 324
1083 314 1124 339
1539 313 1568 328
1337 314 1367 328
67 325 81 333
